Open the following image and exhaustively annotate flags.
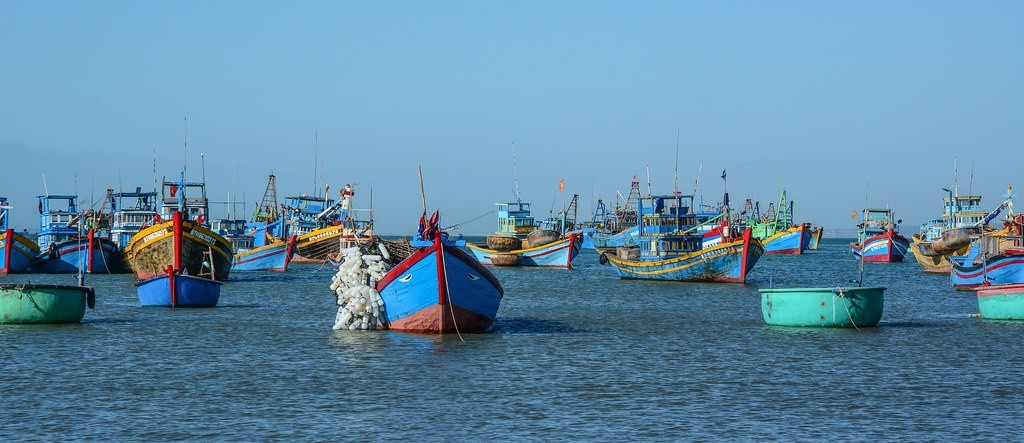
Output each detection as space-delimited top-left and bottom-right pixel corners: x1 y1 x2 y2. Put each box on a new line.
722 169 726 181
560 180 564 190
852 210 857 219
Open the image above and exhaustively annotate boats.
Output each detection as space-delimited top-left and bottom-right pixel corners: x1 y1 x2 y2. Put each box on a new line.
0 284 95 326
377 209 504 334
976 284 1023 321
539 162 823 254
0 116 374 279
135 266 221 308
467 144 584 269
593 125 765 283
850 207 909 263
908 154 1024 291
759 287 885 328
